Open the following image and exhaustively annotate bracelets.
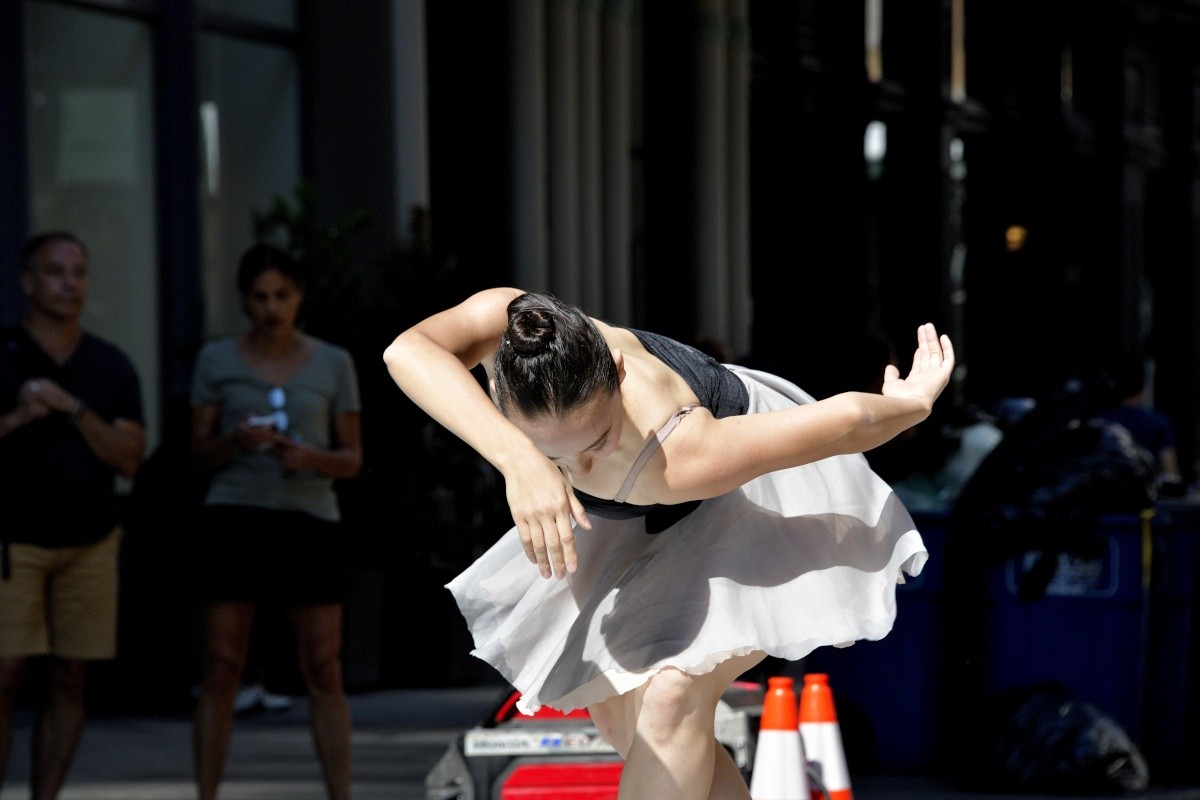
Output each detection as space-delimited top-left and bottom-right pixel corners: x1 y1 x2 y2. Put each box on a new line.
72 399 87 420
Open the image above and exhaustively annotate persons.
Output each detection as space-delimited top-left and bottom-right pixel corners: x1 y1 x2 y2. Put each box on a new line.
189 243 363 800
1 231 148 800
1096 369 1180 483
379 285 955 800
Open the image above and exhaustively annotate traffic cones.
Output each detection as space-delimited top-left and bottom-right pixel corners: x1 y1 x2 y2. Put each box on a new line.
746 676 813 800
798 673 855 800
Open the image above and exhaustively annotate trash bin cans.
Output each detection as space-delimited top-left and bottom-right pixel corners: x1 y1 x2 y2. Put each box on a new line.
757 495 1200 800
